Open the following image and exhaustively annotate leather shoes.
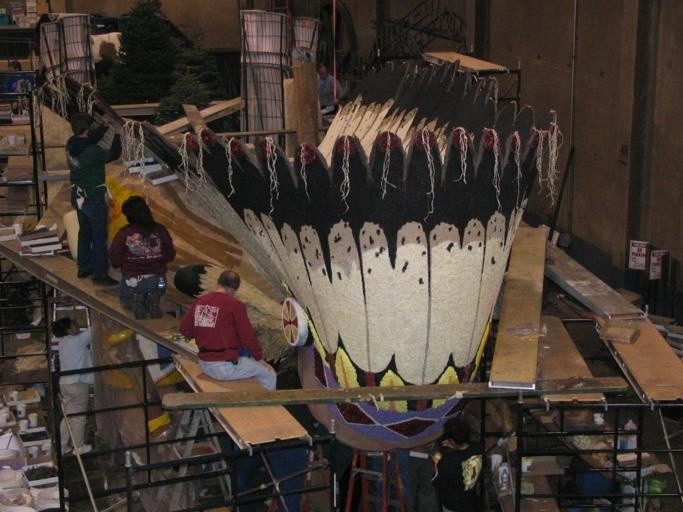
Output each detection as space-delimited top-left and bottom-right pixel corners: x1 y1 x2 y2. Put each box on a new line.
77 270 93 277
93 275 118 285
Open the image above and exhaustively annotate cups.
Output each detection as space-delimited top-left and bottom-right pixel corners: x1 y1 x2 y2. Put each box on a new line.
491 454 503 471
27 447 39 459
593 414 605 425
40 443 53 456
16 404 27 419
28 413 39 428
9 391 20 400
17 420 30 430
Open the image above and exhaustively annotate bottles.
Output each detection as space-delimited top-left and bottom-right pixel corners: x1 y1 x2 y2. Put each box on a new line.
157 277 167 288
497 462 511 493
625 419 638 450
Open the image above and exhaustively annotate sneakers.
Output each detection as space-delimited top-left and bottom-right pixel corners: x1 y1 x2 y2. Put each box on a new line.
148 291 161 317
73 444 92 456
62 446 72 456
133 291 146 320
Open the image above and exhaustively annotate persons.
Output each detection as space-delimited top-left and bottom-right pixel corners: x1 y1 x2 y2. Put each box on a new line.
92 39 117 79
64 111 122 286
178 270 277 391
4 60 34 89
315 62 343 116
108 196 176 320
50 316 94 456
428 417 478 512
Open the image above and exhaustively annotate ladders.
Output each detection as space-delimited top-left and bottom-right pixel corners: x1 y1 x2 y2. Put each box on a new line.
156 408 233 512
345 446 405 512
